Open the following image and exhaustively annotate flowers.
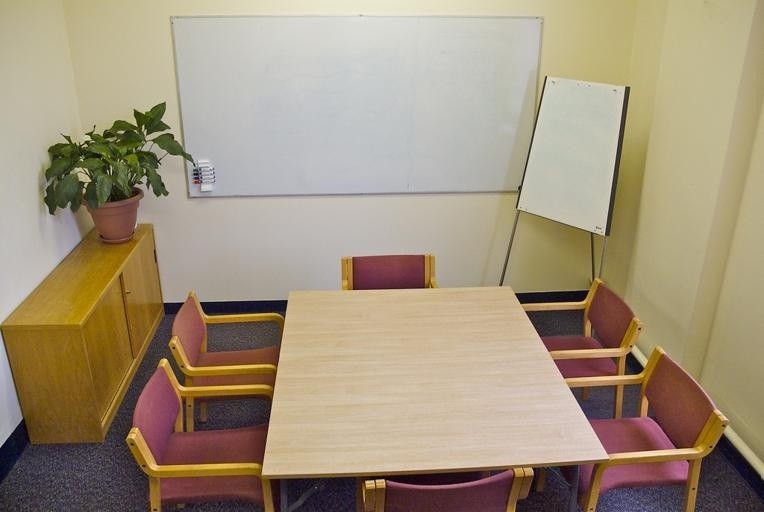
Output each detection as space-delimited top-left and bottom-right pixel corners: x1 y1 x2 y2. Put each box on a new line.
44 102 197 216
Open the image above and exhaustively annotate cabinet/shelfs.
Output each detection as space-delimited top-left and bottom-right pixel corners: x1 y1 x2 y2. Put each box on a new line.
0 223 166 444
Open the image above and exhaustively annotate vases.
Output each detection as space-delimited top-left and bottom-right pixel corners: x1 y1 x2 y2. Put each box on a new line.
81 189 143 243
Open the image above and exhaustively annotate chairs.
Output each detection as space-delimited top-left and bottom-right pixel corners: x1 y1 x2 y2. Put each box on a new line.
522 278 644 421
357 467 536 512
342 255 437 289
169 290 286 431
537 348 728 512
126 359 276 512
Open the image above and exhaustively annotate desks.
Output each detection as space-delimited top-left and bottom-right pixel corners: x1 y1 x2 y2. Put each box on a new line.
262 286 610 512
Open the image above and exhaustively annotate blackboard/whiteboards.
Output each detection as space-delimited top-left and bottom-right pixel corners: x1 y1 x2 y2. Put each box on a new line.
170 16 544 199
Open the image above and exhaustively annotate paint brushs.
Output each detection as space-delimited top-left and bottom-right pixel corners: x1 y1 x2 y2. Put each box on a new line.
192 176 215 180
192 180 215 184
193 167 214 172
191 172 215 176
